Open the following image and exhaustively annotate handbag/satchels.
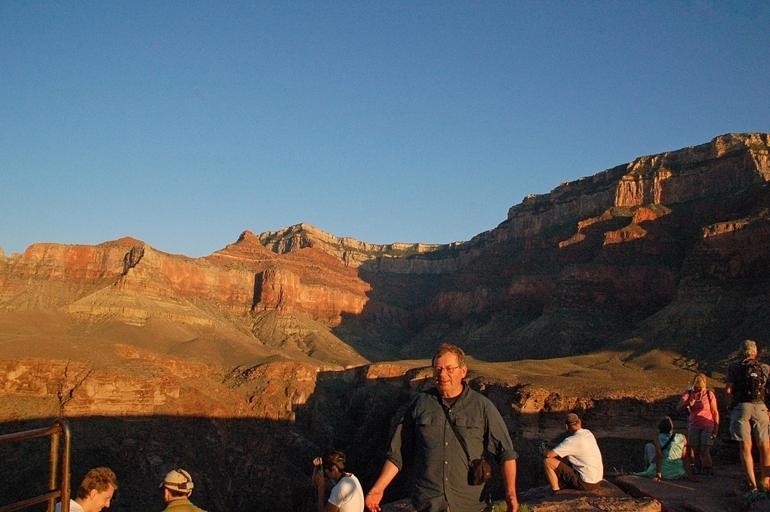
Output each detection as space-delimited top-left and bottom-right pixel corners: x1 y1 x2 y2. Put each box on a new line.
441 402 501 486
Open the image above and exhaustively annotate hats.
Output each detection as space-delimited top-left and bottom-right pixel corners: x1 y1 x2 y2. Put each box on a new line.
158 468 194 494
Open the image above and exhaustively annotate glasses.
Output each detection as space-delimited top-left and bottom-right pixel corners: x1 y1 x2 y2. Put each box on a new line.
435 366 458 373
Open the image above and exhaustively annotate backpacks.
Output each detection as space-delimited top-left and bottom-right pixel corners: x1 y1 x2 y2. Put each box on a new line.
741 359 766 403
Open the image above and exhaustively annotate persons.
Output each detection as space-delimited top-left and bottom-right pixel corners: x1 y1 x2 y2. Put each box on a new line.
54 465 121 512
312 450 365 512
366 344 519 512
538 413 605 496
643 414 694 483
675 374 719 473
724 341 770 495
158 469 209 512
